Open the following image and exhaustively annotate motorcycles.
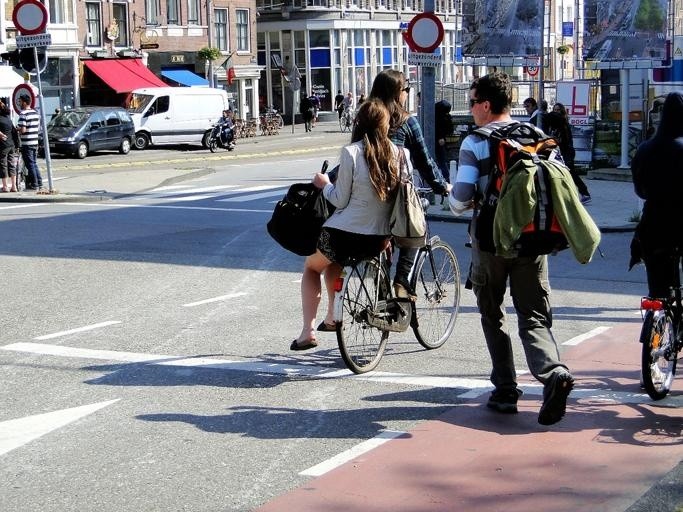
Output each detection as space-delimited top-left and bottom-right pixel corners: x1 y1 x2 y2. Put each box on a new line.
209 123 235 154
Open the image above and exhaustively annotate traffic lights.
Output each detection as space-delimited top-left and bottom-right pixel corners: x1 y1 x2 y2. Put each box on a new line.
0 49 22 71
19 49 46 72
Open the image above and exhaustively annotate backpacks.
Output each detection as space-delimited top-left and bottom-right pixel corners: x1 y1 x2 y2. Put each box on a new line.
470 122 564 257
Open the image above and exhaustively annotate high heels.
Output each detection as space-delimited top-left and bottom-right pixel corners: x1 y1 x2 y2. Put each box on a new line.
393 274 418 299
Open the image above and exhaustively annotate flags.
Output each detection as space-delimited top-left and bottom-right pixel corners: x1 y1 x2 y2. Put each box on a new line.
221 57 236 86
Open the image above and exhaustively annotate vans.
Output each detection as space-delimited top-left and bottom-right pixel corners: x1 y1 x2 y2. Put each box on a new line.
36 106 135 158
123 87 228 149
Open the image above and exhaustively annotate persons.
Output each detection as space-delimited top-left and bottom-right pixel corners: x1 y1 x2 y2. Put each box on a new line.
51 108 60 119
335 90 365 126
369 69 453 299
0 100 21 192
523 98 592 205
448 72 575 425
299 90 319 132
216 110 235 145
416 92 454 196
290 99 413 351
630 90 682 296
15 94 44 193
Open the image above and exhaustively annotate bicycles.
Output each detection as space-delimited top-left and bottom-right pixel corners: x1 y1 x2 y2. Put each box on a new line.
229 106 284 137
634 285 682 401
331 178 462 374
12 144 25 191
339 109 352 133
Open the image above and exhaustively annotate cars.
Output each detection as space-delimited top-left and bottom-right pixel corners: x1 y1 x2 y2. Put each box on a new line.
446 111 609 174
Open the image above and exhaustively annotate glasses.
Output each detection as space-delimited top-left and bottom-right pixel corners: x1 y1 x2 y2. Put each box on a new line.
401 86 410 93
468 98 479 107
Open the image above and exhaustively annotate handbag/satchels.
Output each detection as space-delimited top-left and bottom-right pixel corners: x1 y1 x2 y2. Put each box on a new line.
266 160 330 255
389 179 428 248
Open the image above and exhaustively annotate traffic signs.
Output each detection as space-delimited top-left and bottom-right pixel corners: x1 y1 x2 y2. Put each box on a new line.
526 59 537 76
561 21 574 38
408 54 443 67
12 34 51 49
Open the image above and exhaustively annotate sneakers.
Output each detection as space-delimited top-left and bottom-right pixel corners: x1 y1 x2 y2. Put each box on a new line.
538 374 571 426
581 195 591 203
486 390 522 413
641 363 666 389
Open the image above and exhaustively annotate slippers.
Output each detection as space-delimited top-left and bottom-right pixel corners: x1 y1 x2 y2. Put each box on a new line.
290 340 319 350
317 322 337 332
